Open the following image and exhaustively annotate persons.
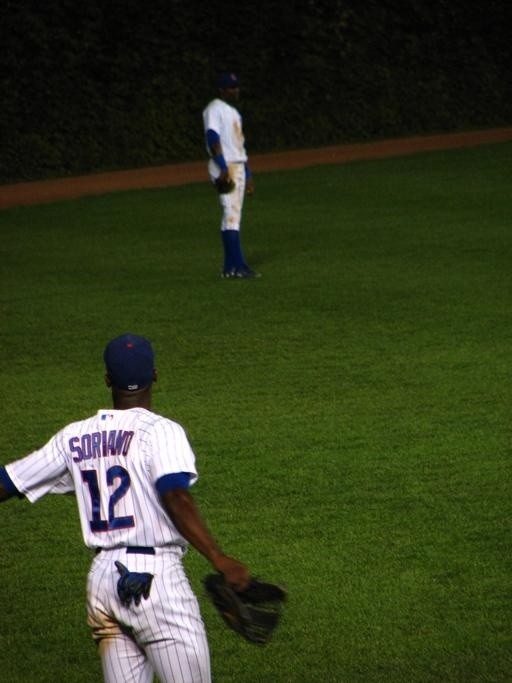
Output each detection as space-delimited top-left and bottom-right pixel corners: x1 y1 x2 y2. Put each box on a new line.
0 332 249 683
203 67 264 278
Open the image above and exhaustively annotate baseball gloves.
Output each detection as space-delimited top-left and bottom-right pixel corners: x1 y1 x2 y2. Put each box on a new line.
206 573 286 644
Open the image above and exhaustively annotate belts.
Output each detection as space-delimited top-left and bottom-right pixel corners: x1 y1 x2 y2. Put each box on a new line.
95 547 154 554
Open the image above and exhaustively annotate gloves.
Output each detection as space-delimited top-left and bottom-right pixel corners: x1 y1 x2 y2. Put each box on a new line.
115 561 153 606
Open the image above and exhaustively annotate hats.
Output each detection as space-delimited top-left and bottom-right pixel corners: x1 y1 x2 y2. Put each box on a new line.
105 334 153 391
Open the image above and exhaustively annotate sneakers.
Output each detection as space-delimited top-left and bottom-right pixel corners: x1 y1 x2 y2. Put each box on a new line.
221 266 261 277
